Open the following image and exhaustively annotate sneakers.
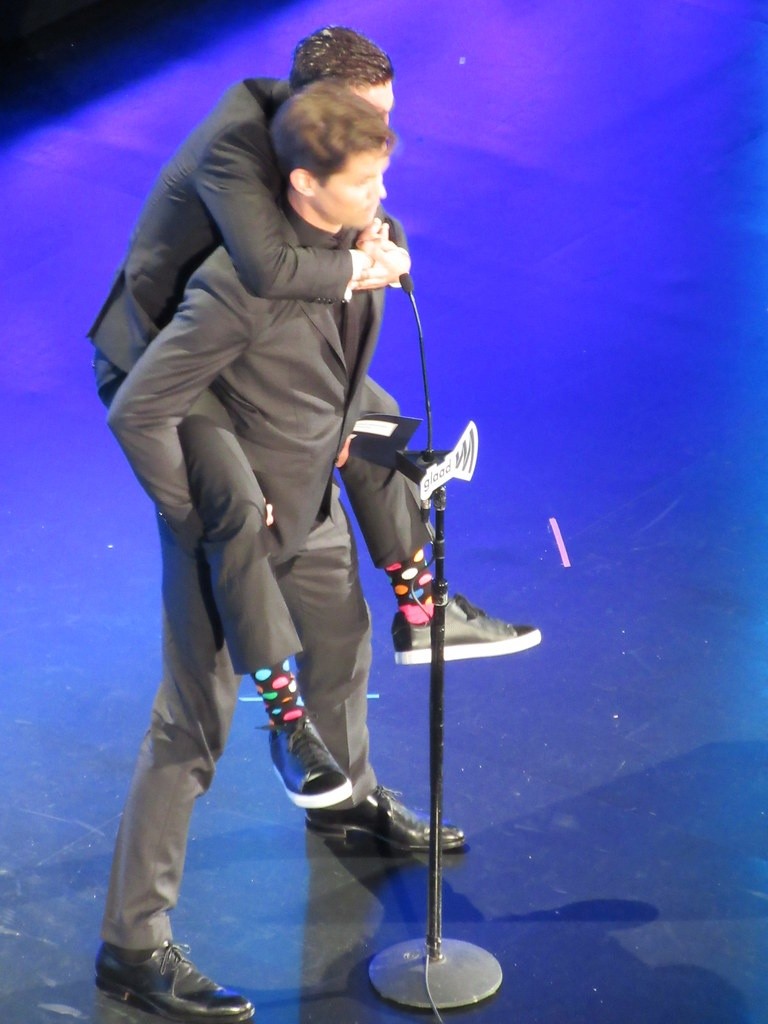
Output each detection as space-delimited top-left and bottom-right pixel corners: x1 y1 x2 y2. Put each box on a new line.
257 711 352 808
391 590 541 664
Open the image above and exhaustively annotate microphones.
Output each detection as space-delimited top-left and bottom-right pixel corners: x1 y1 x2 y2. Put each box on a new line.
399 273 439 464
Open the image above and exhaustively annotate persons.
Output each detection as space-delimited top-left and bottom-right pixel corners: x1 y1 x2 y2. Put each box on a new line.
96 80 464 1024
83 26 543 812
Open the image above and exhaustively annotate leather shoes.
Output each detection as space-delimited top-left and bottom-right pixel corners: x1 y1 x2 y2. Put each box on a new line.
93 939 256 1024
305 779 465 850
